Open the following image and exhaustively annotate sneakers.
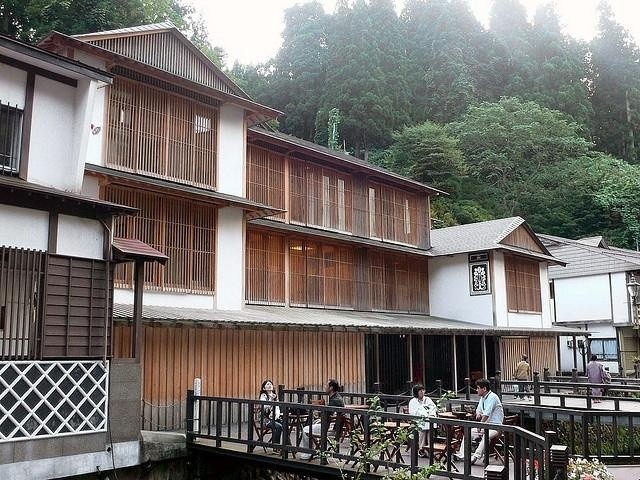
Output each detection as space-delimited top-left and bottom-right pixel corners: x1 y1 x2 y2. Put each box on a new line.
453 453 477 465
300 452 319 460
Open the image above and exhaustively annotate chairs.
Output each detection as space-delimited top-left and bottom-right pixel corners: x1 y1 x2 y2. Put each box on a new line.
253 398 518 480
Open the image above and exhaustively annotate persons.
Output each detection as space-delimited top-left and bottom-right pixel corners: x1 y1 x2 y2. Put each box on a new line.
513 354 532 400
453 377 504 465
257 379 283 454
295 378 346 461
585 355 608 403
408 383 438 458
